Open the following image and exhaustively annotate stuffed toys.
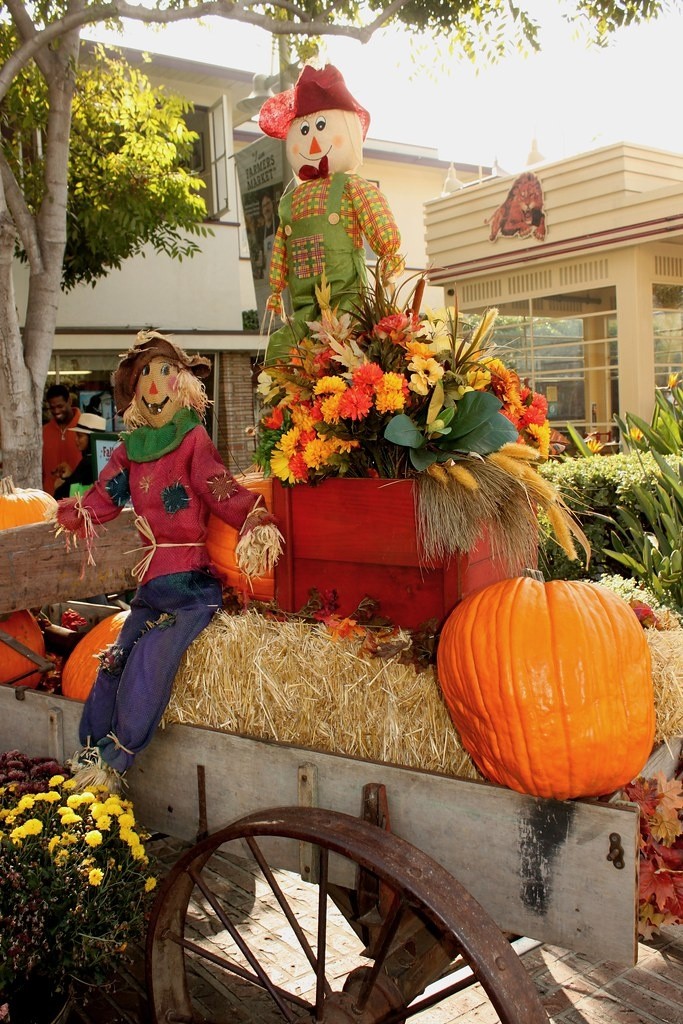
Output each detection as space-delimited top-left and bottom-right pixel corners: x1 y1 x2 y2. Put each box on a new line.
259 60 406 373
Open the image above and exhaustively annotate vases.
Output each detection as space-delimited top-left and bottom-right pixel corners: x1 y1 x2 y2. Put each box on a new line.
267 476 541 632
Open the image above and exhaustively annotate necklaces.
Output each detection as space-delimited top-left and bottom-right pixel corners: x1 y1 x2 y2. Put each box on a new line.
59 426 67 441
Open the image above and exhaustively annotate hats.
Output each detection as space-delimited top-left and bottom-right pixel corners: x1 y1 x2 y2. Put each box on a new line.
67 412 107 435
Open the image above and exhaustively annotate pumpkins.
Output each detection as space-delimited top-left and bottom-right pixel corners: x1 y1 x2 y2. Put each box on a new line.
437 567 655 802
0 476 58 532
0 608 45 690
207 471 275 603
61 609 131 701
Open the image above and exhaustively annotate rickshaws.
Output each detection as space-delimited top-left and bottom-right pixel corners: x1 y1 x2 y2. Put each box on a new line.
0 506 683 1024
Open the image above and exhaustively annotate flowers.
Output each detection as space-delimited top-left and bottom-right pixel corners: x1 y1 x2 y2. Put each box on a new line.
242 253 598 577
0 750 164 1024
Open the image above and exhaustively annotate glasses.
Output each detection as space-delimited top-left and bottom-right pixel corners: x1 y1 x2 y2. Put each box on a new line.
262 202 273 210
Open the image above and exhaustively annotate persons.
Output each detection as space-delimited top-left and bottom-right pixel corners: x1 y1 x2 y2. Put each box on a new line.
84 395 103 416
258 190 281 280
42 328 285 800
53 411 107 502
42 384 81 497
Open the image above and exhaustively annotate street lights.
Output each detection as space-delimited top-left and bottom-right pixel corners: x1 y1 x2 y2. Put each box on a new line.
235 8 295 320
